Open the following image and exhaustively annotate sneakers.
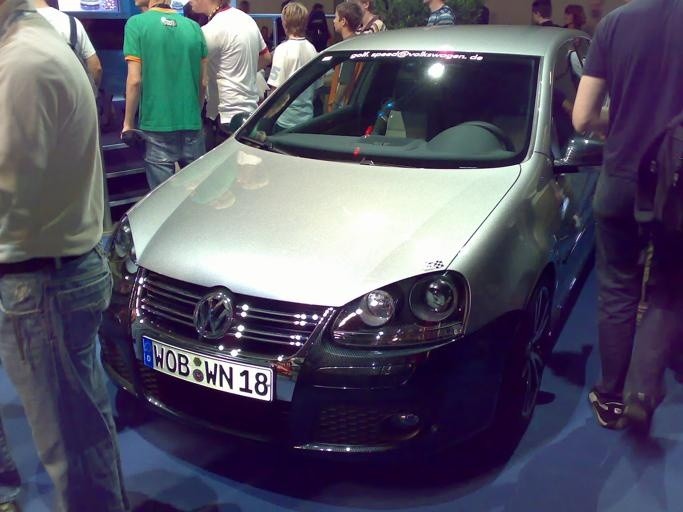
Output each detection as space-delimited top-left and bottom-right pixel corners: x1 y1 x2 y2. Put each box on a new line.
590 387 652 437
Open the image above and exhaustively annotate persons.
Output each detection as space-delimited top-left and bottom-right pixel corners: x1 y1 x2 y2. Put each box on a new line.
266 4 324 135
28 0 104 103
120 0 208 190
564 3 586 30
572 0 682 439
1 1 130 512
329 3 370 112
421 0 457 30
188 0 272 146
532 0 566 28
352 0 388 35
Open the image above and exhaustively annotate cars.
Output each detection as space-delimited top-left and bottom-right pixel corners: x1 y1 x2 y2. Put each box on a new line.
99 26 605 485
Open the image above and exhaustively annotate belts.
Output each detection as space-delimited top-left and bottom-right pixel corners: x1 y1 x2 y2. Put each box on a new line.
0 252 86 275
